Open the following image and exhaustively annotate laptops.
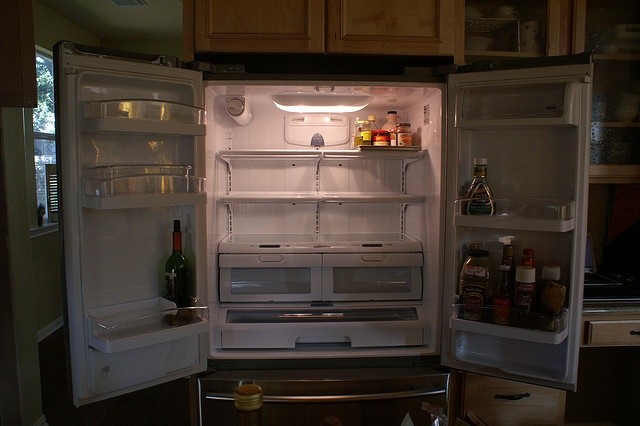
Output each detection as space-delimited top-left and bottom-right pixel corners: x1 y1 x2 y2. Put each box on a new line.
584 232 623 289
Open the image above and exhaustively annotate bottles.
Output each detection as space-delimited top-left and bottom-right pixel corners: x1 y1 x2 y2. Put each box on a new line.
368 115 379 130
534 264 562 331
469 243 480 249
501 245 516 279
522 248 536 268
490 264 512 325
233 385 264 426
164 220 191 325
381 111 402 132
511 266 536 327
461 156 496 217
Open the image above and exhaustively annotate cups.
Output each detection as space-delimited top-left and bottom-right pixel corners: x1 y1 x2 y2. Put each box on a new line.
493 5 520 19
519 19 541 52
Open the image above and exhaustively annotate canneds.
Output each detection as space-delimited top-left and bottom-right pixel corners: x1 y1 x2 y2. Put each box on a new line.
514 265 536 307
397 123 413 146
390 130 396 146
372 129 390 147
355 121 371 145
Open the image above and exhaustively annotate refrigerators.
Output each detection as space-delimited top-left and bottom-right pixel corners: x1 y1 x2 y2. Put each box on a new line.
50 39 595 425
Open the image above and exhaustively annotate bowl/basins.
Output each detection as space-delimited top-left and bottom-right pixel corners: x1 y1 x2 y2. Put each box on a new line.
610 93 639 122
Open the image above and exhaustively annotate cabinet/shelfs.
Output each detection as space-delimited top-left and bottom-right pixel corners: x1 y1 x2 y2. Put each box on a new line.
572 0 640 185
0 0 38 108
180 0 464 69
579 269 640 348
464 0 573 66
447 368 568 426
